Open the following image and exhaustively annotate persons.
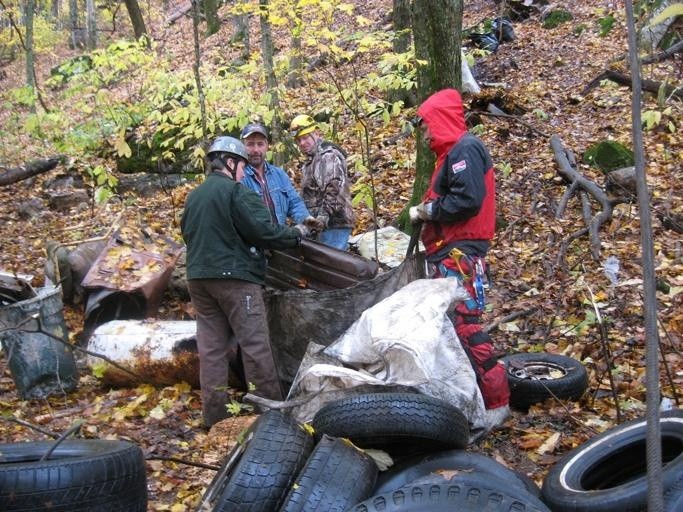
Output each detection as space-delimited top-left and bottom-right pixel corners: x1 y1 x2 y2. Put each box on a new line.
409 90 511 429
180 136 312 437
240 125 314 226
290 116 353 251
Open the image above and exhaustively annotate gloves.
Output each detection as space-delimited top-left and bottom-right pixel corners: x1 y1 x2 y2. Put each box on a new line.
317 215 329 229
294 223 311 238
408 200 430 225
305 215 323 230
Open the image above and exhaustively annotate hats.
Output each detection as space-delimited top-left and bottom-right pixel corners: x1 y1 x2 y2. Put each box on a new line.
240 125 268 139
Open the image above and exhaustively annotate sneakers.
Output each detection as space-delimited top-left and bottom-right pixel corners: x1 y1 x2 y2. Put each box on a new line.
485 403 510 432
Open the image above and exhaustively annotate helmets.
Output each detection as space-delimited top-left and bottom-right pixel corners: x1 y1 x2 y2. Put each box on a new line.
206 137 249 163
290 114 318 140
